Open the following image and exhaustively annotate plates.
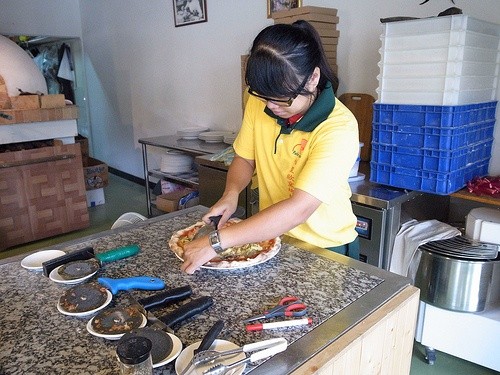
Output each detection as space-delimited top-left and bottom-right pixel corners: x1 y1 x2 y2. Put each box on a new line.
116 333 183 368
57 284 112 317
22 250 66 272
160 149 193 173
170 218 281 269
49 258 100 285
176 127 237 145
86 313 147 340
175 340 246 374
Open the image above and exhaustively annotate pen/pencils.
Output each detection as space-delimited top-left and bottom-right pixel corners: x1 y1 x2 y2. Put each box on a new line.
246 318 313 331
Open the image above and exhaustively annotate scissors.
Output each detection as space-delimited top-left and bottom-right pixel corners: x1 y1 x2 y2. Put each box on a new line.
245 297 307 322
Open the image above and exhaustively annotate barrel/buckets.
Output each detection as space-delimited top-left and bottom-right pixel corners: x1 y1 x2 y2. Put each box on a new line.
417 250 500 313
349 141 364 177
111 212 146 229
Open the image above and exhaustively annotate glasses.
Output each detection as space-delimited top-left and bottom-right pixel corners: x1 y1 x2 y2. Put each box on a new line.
248 70 310 107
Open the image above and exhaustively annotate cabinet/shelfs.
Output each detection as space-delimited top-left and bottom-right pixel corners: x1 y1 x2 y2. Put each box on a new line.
137 134 259 220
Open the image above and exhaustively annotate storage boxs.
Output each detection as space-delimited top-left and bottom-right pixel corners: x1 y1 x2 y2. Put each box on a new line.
86 188 105 207
369 101 498 195
376 13 500 106
272 7 340 74
157 187 198 212
75 134 89 159
84 157 109 191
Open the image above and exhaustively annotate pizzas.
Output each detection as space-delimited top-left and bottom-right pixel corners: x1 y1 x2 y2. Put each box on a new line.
168 218 281 268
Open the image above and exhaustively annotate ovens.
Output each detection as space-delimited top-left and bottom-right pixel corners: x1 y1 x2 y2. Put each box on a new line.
351 203 386 268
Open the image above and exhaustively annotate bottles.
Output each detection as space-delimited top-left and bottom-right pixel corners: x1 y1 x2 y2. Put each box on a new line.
117 337 153 375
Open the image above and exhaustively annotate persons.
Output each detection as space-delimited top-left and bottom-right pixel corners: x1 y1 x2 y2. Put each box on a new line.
184 0 202 18
180 20 359 275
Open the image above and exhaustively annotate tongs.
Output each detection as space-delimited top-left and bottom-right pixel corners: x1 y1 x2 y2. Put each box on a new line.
191 337 288 374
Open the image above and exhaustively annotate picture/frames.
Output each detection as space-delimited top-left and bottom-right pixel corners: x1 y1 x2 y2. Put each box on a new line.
173 0 207 27
267 0 302 18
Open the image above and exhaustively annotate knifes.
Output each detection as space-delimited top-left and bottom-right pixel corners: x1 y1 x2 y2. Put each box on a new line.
191 215 222 240
180 320 224 375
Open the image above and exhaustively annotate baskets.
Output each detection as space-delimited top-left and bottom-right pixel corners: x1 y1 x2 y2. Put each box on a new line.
111 212 148 230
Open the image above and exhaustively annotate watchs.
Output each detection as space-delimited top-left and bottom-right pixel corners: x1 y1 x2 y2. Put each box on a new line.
208 229 223 254
